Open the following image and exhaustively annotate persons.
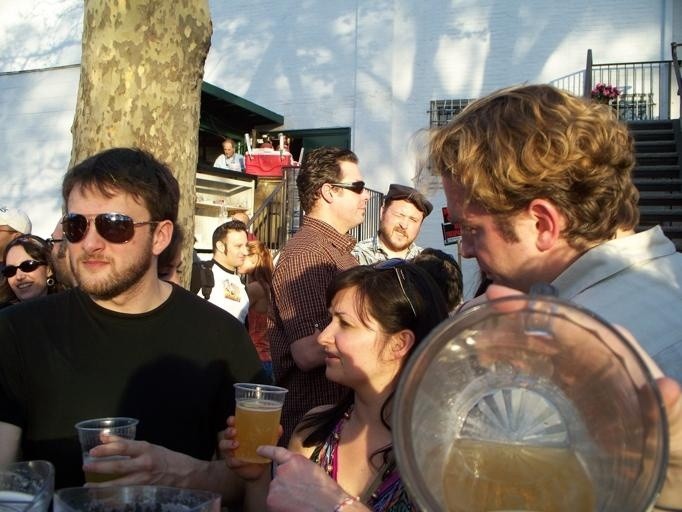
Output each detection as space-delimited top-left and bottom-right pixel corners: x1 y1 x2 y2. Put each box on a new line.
410 84 681 385
0 147 266 509
351 183 433 265
216 258 445 511
1 206 279 387
411 249 464 318
212 138 244 172
269 144 371 476
480 282 682 511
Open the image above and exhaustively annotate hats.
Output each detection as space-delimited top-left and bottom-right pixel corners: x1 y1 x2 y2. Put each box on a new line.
387 184 432 217
0 208 32 235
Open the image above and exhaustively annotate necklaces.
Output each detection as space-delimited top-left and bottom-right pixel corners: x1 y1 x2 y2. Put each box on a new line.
326 405 391 499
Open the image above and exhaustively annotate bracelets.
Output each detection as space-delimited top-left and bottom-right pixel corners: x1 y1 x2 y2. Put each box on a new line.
334 492 360 511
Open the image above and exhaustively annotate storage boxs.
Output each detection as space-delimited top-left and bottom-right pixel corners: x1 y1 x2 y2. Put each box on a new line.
245 154 291 177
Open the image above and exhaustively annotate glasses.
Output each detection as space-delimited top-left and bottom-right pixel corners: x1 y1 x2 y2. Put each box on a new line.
45 237 64 247
330 181 365 194
373 257 419 317
61 212 160 244
4 259 45 279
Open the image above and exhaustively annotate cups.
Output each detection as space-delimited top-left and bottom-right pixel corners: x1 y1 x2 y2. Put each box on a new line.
0 457 55 510
390 297 670 511
55 481 223 511
232 380 289 466
74 415 138 484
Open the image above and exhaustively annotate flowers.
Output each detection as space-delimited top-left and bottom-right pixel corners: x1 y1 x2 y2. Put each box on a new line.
592 82 621 106
